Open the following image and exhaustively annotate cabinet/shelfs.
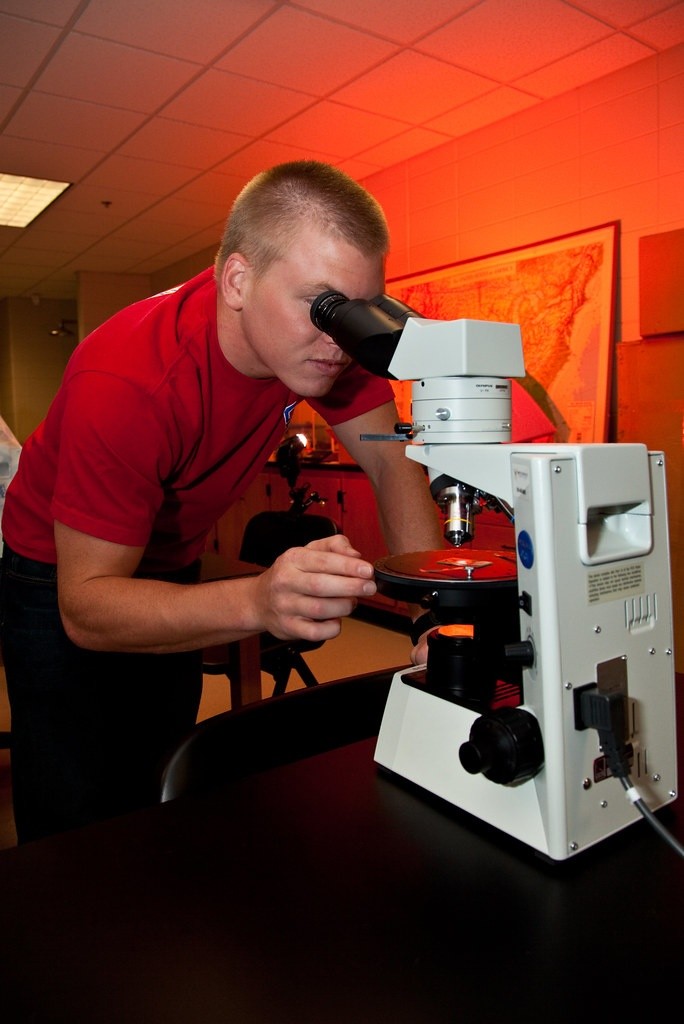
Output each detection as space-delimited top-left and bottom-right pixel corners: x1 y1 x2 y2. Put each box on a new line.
205 452 518 617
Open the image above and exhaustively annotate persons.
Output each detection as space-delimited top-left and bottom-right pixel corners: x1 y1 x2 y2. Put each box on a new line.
0 159 447 846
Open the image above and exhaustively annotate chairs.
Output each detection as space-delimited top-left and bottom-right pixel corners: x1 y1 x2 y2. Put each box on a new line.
200 509 338 696
157 661 417 800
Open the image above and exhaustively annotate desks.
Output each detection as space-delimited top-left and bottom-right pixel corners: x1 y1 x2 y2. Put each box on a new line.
0 735 684 1023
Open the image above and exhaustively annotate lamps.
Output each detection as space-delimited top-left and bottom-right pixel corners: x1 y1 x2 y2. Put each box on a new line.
48 317 77 337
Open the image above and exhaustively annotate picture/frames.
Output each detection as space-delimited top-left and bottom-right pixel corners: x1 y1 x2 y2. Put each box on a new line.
385 220 620 443
